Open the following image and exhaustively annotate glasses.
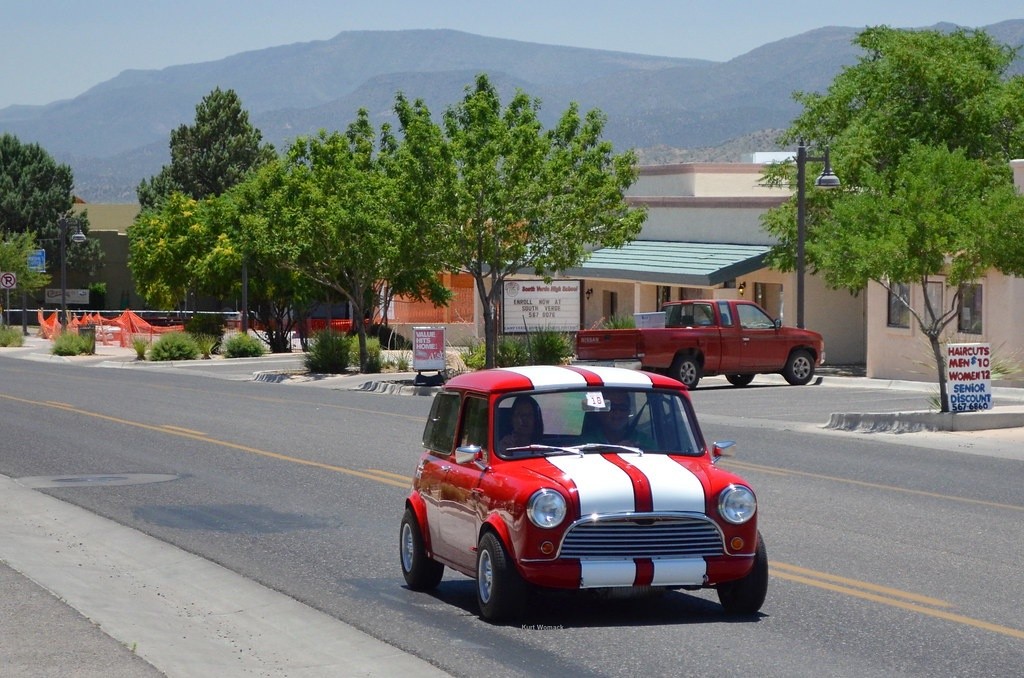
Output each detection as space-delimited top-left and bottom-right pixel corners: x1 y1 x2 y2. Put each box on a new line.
610 403 630 412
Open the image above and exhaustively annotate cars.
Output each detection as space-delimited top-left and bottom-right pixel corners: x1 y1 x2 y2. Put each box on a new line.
398 362 770 621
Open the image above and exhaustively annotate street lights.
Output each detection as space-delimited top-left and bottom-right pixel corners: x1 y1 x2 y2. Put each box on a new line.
791 139 842 330
58 218 86 336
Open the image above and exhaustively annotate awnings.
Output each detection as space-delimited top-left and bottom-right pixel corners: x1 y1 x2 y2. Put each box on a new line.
470 237 778 286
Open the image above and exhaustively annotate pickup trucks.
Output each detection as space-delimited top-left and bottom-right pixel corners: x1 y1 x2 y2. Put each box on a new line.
573 297 827 391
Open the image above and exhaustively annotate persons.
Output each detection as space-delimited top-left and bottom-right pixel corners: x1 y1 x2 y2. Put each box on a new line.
576 388 659 453
499 395 560 456
